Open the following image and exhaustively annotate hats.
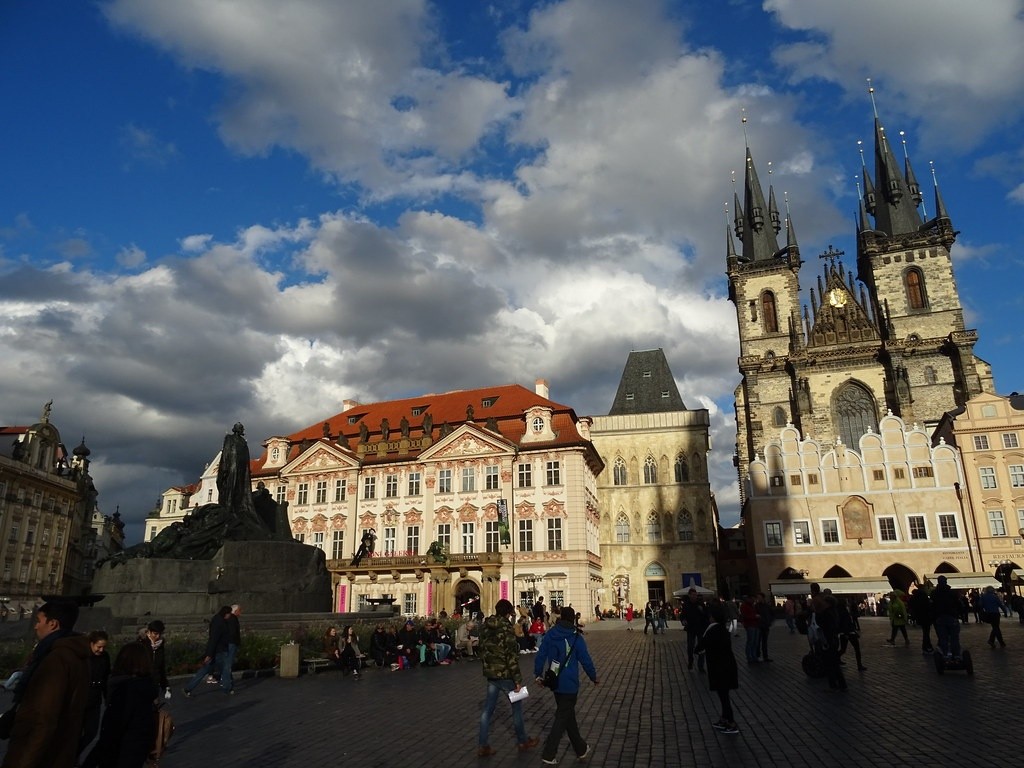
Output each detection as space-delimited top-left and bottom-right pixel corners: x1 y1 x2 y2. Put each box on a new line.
560 607 574 627
406 620 415 626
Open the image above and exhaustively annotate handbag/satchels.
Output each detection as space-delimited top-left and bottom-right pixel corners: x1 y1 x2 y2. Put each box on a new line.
802 651 826 679
544 669 559 691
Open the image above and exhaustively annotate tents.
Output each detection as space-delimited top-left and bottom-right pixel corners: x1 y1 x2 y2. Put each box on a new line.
672 585 714 609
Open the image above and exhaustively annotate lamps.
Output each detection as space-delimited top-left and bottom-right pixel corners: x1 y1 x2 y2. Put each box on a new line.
420 560 428 566
858 537 863 546
487 553 491 561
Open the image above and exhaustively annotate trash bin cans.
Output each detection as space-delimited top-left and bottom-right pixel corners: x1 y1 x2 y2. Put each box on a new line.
279 639 301 678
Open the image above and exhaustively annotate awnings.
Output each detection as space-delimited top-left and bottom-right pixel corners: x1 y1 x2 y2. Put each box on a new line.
769 576 893 594
929 577 1002 589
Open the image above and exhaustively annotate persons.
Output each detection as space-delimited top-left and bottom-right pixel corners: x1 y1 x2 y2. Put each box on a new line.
438 607 485 623
868 595 889 617
667 588 777 676
181 604 241 697
694 608 740 734
624 604 633 630
192 422 265 517
595 605 604 620
784 582 868 692
299 405 500 454
369 618 480 672
0 600 173 768
511 596 588 654
907 575 964 657
349 528 375 568
477 599 539 756
534 607 600 764
323 626 362 681
981 586 1008 647
886 592 910 645
959 589 1024 624
644 600 669 635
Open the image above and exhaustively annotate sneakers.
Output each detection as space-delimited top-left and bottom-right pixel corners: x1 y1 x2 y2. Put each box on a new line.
721 725 739 734
711 716 725 729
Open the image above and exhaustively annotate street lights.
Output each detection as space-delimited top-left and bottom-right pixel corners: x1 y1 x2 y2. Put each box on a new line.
788 569 809 609
523 574 543 606
988 559 1012 605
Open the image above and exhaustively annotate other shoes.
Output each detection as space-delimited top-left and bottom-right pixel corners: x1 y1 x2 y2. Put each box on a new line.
541 758 560 765
478 745 496 756
858 664 867 671
763 658 773 662
519 736 540 753
999 641 1006 649
577 744 593 759
688 664 693 670
905 638 910 648
520 649 527 654
207 675 219 684
440 659 451 665
531 649 537 653
224 689 240 696
526 649 531 653
988 640 996 648
184 689 191 702
887 638 895 645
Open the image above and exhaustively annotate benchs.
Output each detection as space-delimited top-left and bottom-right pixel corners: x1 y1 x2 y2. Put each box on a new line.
303 653 367 677
